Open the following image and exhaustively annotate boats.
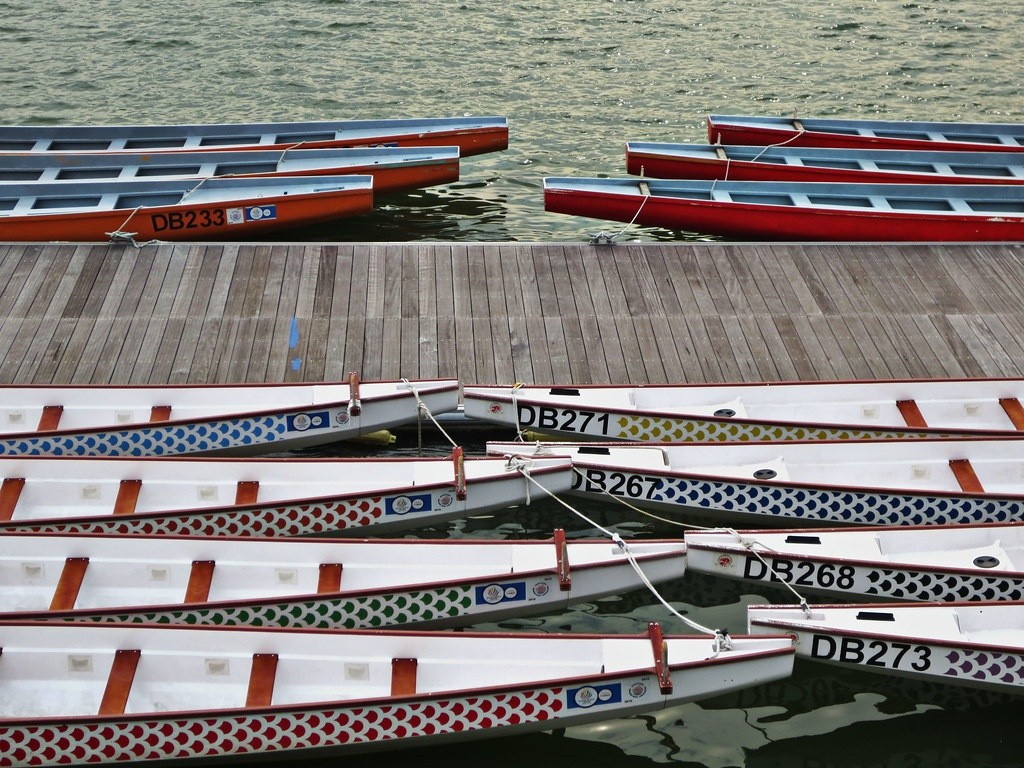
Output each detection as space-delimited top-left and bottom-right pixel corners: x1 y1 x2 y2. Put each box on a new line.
0 115 509 159
0 173 375 243
708 113 1024 152
0 144 461 194
542 174 1024 241
684 520 1024 603
481 437 1024 524
625 139 1024 186
747 599 1024 697
0 617 797 768
0 449 575 539
1 378 461 460
0 528 688 630
462 376 1024 440
